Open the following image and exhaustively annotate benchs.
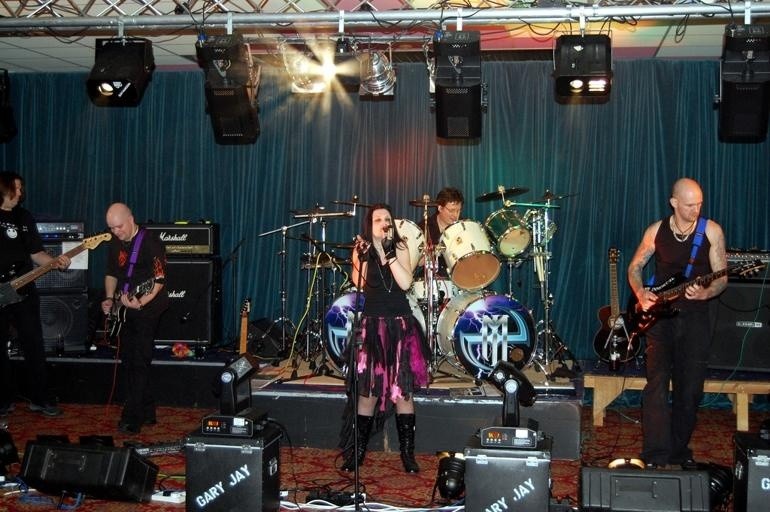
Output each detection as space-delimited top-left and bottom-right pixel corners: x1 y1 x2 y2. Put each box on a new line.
583 372 770 431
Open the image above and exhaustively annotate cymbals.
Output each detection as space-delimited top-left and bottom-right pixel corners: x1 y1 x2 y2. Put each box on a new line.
333 242 357 250
533 195 575 205
476 187 528 202
294 208 345 218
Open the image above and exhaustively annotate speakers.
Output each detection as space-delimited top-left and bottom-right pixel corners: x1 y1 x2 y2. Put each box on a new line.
33 220 90 347
706 281 770 373
21 434 159 503
142 256 225 352
732 431 770 512
464 435 552 511
184 429 284 512
579 468 711 511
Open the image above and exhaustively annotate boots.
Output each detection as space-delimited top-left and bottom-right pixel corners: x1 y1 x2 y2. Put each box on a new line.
396 413 419 473
341 415 374 472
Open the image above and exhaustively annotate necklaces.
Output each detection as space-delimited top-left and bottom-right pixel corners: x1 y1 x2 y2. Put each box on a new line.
128 226 138 240
669 214 696 244
671 215 696 239
374 258 394 293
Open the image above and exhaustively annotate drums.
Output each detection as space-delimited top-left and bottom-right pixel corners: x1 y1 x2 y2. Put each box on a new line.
437 288 538 381
485 208 531 257
393 219 426 272
322 288 367 373
438 219 500 291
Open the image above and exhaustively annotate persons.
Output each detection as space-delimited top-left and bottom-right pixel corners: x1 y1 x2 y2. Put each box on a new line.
1 171 71 417
337 203 433 474
628 177 728 466
414 187 467 280
100 201 169 434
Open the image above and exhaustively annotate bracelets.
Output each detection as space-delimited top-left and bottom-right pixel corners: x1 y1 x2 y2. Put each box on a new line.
388 258 399 266
105 296 115 303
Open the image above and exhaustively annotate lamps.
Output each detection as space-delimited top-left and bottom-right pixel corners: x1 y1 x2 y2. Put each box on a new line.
553 34 613 103
358 50 397 99
713 26 770 143
288 39 357 96
87 38 156 108
195 36 260 147
428 31 489 143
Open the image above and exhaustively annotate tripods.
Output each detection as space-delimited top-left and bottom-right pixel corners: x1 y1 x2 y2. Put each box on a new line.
535 211 582 383
257 225 346 383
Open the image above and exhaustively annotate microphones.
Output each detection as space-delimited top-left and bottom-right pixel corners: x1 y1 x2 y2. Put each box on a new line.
387 224 393 241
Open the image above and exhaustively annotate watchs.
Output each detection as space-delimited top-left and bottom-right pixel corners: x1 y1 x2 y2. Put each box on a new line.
138 300 144 313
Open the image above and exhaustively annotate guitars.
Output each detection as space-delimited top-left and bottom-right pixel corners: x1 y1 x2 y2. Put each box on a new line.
1 233 112 306
593 247 642 364
105 277 156 338
627 258 766 336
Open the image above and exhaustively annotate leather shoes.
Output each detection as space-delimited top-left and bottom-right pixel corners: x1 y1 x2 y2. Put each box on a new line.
119 420 141 434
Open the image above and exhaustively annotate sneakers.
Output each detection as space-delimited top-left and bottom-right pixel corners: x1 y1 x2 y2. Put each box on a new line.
30 401 62 416
0 411 8 429
